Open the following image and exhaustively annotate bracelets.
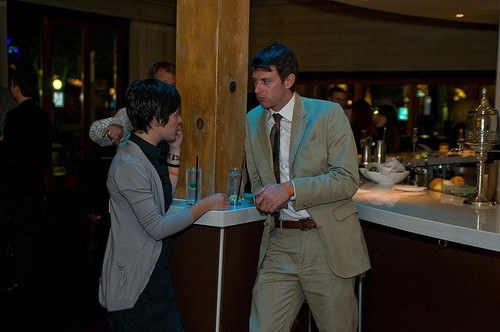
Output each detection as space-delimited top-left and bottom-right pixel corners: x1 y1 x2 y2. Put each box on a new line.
169 170 180 176
167 162 180 168
168 153 180 160
283 182 293 197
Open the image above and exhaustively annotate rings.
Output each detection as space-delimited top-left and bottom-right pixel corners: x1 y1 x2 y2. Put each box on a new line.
224 198 228 201
108 131 111 134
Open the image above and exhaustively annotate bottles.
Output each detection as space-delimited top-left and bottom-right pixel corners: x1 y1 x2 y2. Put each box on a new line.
361 145 372 165
373 141 386 163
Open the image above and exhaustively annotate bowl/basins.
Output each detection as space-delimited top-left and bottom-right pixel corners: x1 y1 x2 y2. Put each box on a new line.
364 169 410 186
359 167 369 179
441 183 477 197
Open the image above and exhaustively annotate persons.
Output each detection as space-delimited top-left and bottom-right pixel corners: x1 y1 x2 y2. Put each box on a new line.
244 43 371 332
327 86 408 153
89 62 232 332
0 69 55 295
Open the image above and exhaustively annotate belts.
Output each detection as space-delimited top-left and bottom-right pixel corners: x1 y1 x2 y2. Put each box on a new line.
275 218 316 231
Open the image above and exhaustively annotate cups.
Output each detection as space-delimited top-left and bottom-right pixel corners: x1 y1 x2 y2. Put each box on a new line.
228 167 245 206
185 167 201 206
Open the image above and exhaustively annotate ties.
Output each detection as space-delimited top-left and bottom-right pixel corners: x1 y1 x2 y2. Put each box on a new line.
270 113 283 221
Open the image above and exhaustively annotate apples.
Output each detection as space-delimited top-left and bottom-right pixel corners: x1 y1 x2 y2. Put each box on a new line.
428 176 464 192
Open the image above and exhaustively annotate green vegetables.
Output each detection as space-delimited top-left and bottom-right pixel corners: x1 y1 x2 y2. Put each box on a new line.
458 185 474 199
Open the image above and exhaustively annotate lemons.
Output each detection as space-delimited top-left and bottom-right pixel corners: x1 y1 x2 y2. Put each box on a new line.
188 182 199 189
229 194 241 200
